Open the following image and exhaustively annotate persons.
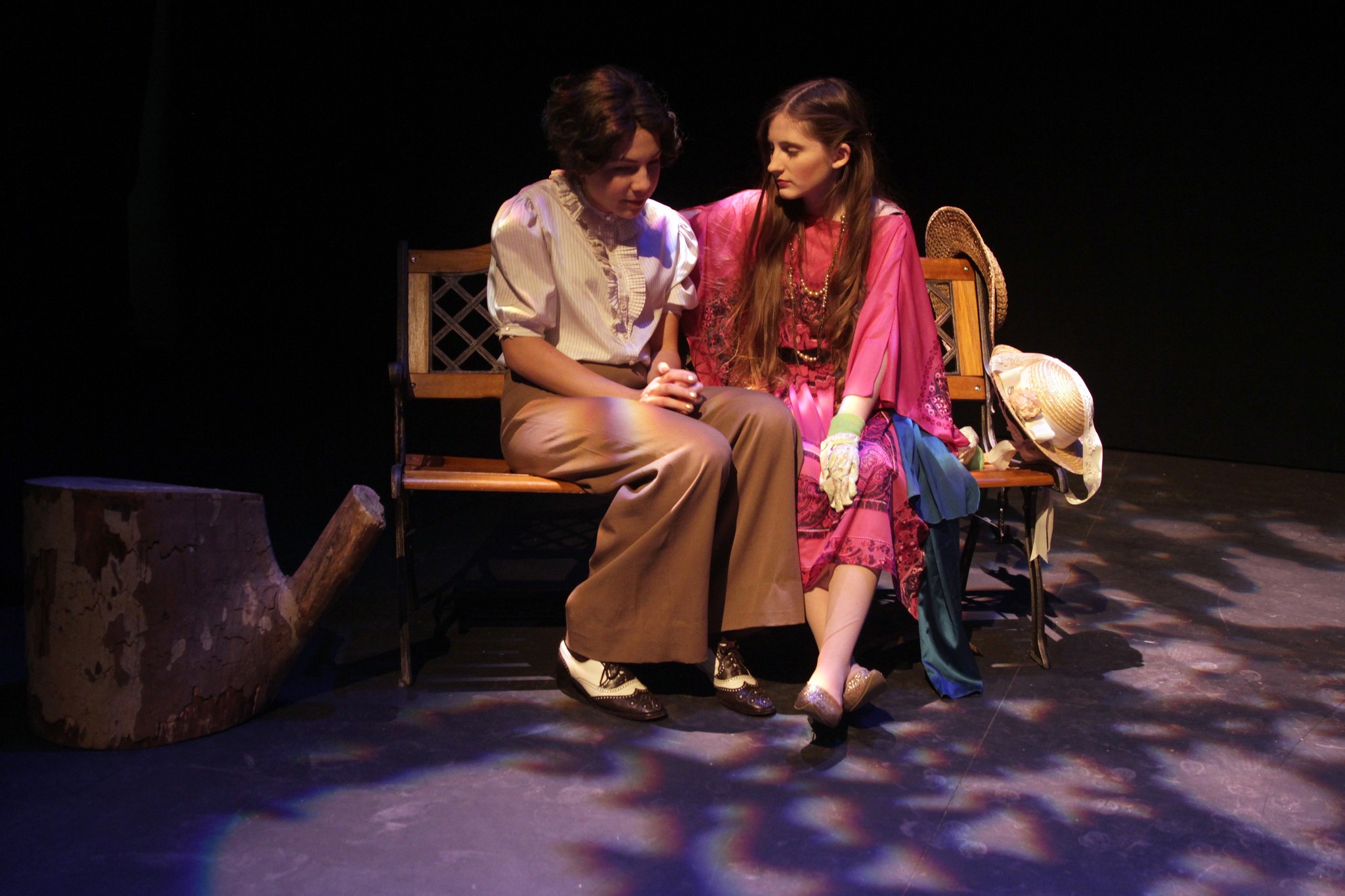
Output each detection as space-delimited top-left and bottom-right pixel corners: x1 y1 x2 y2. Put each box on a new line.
487 68 807 720
674 79 980 725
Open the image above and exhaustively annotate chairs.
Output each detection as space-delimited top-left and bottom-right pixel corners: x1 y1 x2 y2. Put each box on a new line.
878 257 1056 669
389 237 599 688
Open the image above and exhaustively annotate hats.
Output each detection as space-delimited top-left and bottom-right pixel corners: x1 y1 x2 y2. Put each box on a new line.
989 344 1094 475
925 206 1008 355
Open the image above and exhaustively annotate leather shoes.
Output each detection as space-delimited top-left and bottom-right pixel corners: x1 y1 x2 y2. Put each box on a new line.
696 633 777 715
556 637 667 720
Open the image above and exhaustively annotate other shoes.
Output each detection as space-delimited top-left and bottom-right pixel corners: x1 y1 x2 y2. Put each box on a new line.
794 684 842 728
842 669 888 712
